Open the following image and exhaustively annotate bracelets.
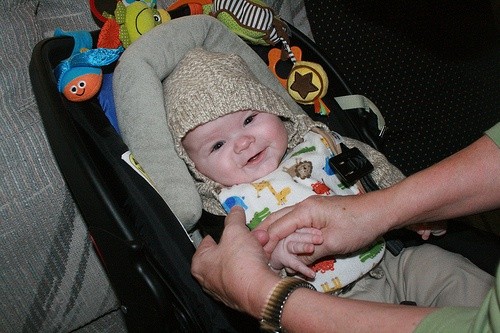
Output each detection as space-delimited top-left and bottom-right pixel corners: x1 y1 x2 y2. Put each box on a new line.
259 275 317 333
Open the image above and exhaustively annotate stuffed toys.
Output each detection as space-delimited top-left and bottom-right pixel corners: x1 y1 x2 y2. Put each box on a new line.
55 0 333 117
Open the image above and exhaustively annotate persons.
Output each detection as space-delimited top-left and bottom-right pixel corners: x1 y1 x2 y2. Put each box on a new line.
161 46 494 312
192 120 500 333
